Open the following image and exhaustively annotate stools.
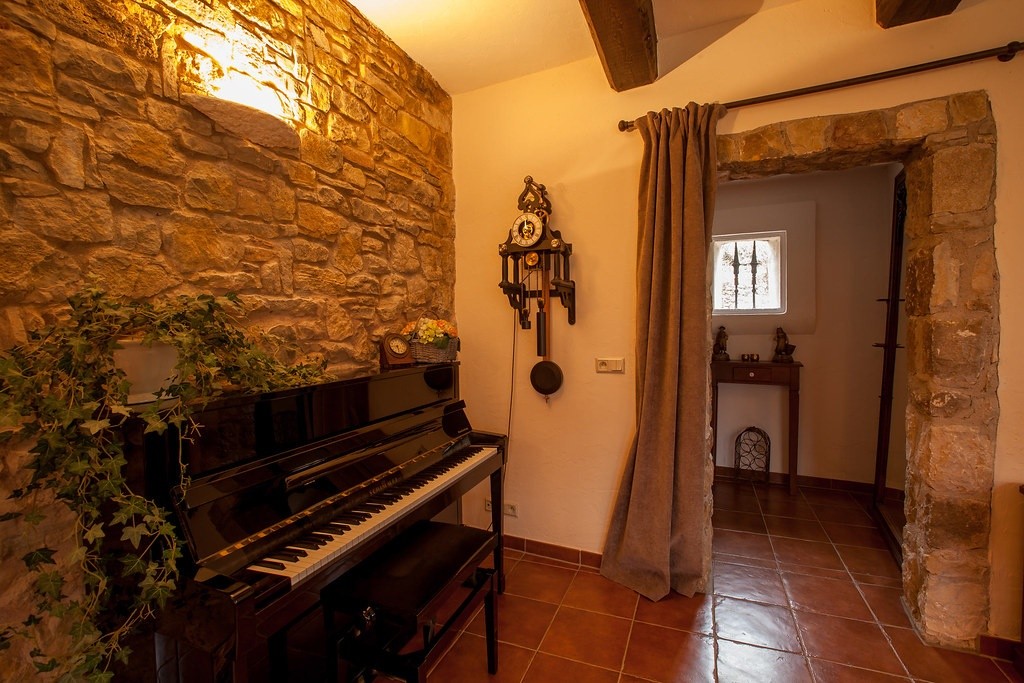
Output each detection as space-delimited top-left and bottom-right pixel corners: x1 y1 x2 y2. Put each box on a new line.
321 519 503 683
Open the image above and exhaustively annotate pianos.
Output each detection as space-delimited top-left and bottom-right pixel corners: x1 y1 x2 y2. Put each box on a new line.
78 359 509 683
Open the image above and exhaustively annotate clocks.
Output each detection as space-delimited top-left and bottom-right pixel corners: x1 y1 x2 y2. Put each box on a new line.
500 176 576 405
380 333 415 371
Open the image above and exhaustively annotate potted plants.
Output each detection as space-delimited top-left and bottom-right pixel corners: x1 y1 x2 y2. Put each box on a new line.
0 284 336 683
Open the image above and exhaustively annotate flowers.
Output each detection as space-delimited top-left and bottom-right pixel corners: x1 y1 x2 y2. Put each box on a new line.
400 318 460 345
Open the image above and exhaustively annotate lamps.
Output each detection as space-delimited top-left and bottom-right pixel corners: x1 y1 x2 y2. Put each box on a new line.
182 92 301 151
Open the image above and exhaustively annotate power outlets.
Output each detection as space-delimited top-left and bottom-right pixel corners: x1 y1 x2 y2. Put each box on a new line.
485 498 519 517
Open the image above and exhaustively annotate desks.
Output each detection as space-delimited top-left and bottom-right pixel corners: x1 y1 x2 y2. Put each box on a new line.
712 360 803 496
